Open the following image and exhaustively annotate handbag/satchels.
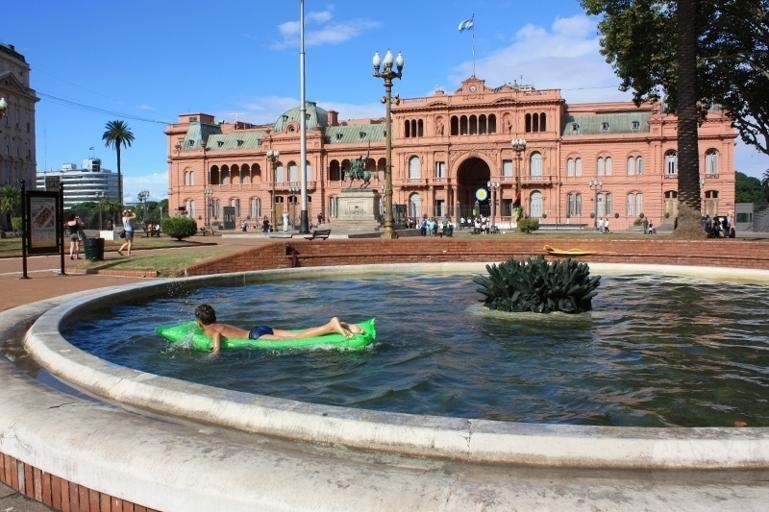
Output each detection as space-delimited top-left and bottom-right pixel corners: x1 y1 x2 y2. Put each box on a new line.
75 229 87 241
118 229 126 238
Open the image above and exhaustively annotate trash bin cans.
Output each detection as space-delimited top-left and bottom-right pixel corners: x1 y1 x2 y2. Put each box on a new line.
444 228 452 236
85 237 105 261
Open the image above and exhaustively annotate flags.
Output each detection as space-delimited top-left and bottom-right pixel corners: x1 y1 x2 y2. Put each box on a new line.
458 13 475 33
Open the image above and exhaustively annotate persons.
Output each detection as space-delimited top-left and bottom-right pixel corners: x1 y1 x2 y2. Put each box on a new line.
598 215 604 233
118 208 136 258
64 213 85 259
147 222 153 232
195 303 365 357
155 223 161 236
317 212 322 224
262 216 269 233
642 217 648 233
241 220 249 232
604 216 610 233
405 212 501 236
704 211 735 240
648 219 654 233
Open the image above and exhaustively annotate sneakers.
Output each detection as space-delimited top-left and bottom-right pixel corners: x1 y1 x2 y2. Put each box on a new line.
128 253 135 257
117 249 124 257
70 255 74 260
77 257 83 259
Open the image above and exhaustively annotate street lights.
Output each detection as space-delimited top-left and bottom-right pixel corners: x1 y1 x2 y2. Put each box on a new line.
0 96 9 121
486 181 502 229
95 191 104 230
587 178 603 230
510 138 526 208
265 148 281 231
201 188 214 229
137 190 150 217
369 46 405 243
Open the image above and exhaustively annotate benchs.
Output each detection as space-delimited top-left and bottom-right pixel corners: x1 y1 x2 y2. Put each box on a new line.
304 229 331 240
